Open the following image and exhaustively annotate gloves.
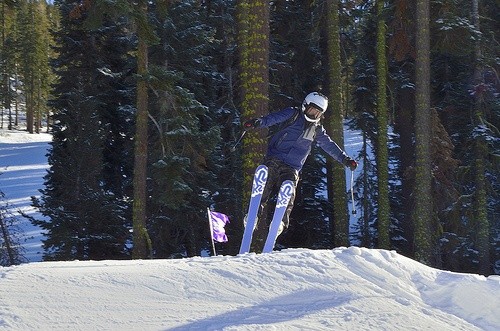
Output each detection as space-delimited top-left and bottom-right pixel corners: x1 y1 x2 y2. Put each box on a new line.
342 156 357 171
241 117 260 128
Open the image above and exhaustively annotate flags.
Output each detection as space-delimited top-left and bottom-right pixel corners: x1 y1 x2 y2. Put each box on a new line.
210 210 230 243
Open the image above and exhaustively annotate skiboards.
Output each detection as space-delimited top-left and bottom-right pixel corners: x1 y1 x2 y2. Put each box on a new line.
262 179 294 254
239 164 269 255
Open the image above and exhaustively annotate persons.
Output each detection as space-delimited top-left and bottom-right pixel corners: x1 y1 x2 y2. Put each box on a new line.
242 91 357 237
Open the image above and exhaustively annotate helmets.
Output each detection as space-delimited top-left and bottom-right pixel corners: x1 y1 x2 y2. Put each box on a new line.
302 92 329 123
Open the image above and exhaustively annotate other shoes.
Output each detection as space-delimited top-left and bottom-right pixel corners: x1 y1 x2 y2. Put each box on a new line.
244 213 258 230
269 220 284 240
